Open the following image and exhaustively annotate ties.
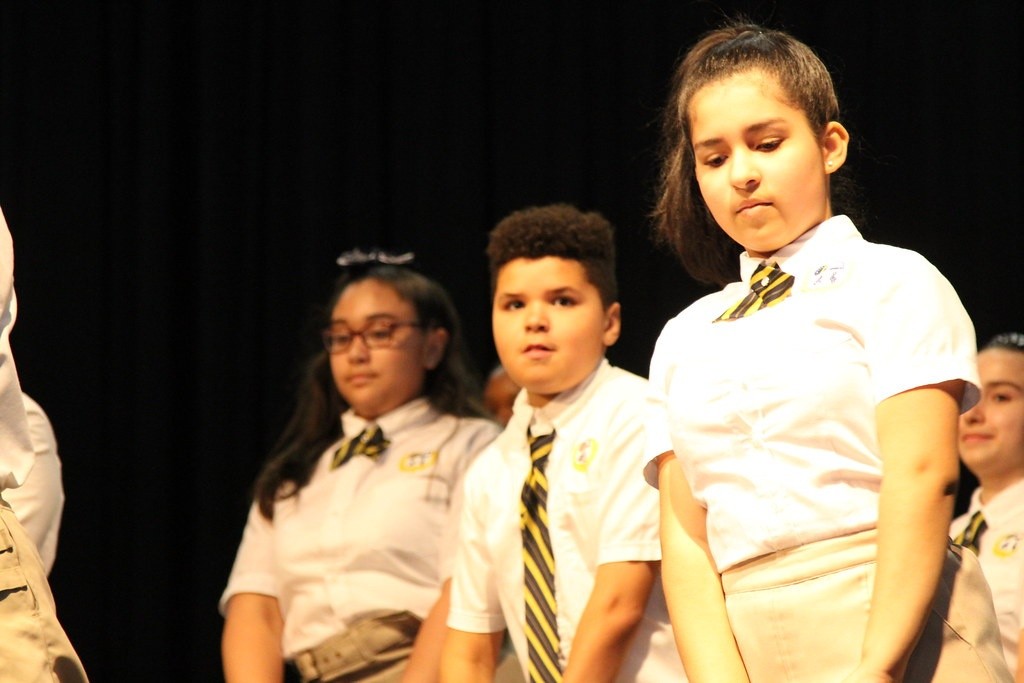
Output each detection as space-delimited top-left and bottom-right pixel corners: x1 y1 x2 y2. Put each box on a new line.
519 425 566 683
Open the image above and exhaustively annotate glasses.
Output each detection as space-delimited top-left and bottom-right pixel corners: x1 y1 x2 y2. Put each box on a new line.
320 321 427 355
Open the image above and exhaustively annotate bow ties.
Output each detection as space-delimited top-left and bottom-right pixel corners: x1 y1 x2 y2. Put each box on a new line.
713 263 795 323
953 512 987 557
331 425 388 468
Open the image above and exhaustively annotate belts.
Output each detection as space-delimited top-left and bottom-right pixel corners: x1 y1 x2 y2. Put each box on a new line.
296 610 419 683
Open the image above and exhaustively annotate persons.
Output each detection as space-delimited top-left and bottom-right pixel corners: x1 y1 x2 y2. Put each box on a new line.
949 333 1024 683
437 205 689 683
483 364 522 428
638 24 985 683
218 250 499 683
